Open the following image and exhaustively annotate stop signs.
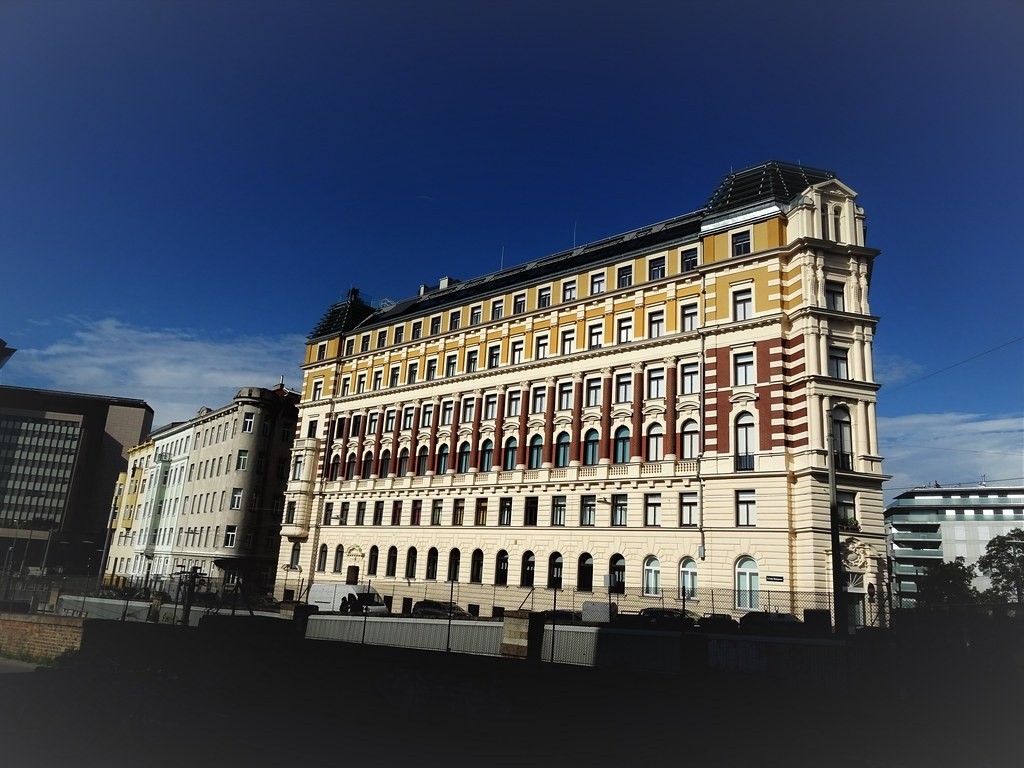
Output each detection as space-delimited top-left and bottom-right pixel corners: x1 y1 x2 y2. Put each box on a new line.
867 583 875 597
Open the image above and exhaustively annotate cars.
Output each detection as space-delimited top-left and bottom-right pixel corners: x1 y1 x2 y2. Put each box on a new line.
412 600 474 621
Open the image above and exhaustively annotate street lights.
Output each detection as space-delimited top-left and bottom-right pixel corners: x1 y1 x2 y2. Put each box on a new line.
0 520 27 602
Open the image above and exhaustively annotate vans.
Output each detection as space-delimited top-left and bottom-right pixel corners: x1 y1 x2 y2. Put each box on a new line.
308 583 389 617
